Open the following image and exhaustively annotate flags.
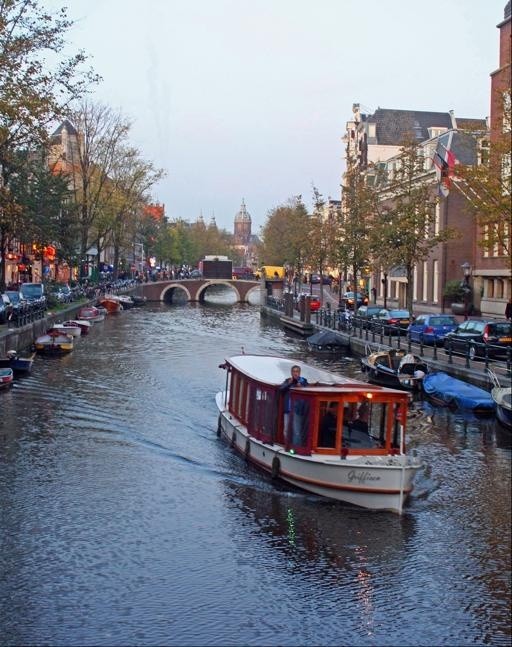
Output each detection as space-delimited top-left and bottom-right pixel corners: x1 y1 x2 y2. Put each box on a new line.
278 363 310 447
435 154 467 183
443 149 466 178
430 160 450 199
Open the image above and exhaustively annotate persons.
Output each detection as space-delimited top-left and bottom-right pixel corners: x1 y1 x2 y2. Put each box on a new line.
318 399 372 448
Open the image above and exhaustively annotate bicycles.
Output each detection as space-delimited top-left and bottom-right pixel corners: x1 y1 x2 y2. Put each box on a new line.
319 307 353 334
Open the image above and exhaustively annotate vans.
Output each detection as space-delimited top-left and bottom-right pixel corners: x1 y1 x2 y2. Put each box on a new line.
232 267 252 278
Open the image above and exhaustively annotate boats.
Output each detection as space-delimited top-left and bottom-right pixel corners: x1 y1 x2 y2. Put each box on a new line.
359 342 511 425
35 295 147 354
0 357 34 389
215 346 428 517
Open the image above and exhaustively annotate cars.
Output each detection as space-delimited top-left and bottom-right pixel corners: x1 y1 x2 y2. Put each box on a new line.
292 292 320 312
338 290 511 360
303 271 332 285
0 282 80 317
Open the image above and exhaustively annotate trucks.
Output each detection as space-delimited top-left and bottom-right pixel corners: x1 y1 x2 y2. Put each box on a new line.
255 265 286 279
